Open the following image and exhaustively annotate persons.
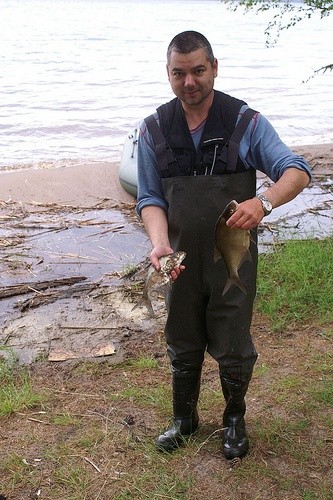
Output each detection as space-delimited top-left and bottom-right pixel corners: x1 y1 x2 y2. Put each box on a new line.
133 29 313 462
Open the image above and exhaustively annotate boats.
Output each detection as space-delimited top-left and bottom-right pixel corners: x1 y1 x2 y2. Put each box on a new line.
117 125 140 197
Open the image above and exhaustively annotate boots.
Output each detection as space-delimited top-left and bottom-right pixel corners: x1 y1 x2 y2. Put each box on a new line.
156 382 201 452
221 377 250 459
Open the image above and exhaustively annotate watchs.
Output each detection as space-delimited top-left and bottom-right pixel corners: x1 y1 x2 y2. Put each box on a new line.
255 193 273 217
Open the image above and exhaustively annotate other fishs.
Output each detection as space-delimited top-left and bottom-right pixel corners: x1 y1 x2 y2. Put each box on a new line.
131 251 187 317
213 199 256 296
